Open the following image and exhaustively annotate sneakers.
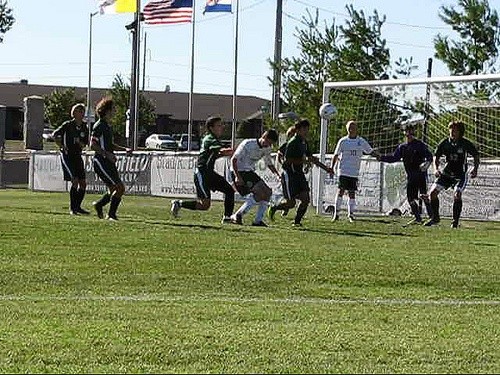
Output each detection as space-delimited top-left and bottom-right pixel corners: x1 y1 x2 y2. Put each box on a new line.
424 215 439 226
294 216 301 224
93 201 103 219
403 217 421 226
450 220 460 228
221 214 240 224
270 207 276 224
70 208 90 216
105 213 119 221
349 216 355 223
252 219 267 226
331 215 339 222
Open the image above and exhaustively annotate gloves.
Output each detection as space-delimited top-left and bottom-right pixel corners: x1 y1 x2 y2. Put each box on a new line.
371 149 379 157
420 162 430 171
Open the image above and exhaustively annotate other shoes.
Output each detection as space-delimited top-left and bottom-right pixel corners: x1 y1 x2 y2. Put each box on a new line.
169 199 181 212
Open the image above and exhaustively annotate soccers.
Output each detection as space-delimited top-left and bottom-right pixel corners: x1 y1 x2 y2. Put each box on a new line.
319 103 338 120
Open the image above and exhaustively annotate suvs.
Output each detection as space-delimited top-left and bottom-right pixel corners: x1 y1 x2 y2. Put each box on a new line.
172 133 199 151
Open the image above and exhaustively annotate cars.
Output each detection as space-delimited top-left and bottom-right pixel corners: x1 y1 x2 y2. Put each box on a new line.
42 128 56 143
145 133 178 152
